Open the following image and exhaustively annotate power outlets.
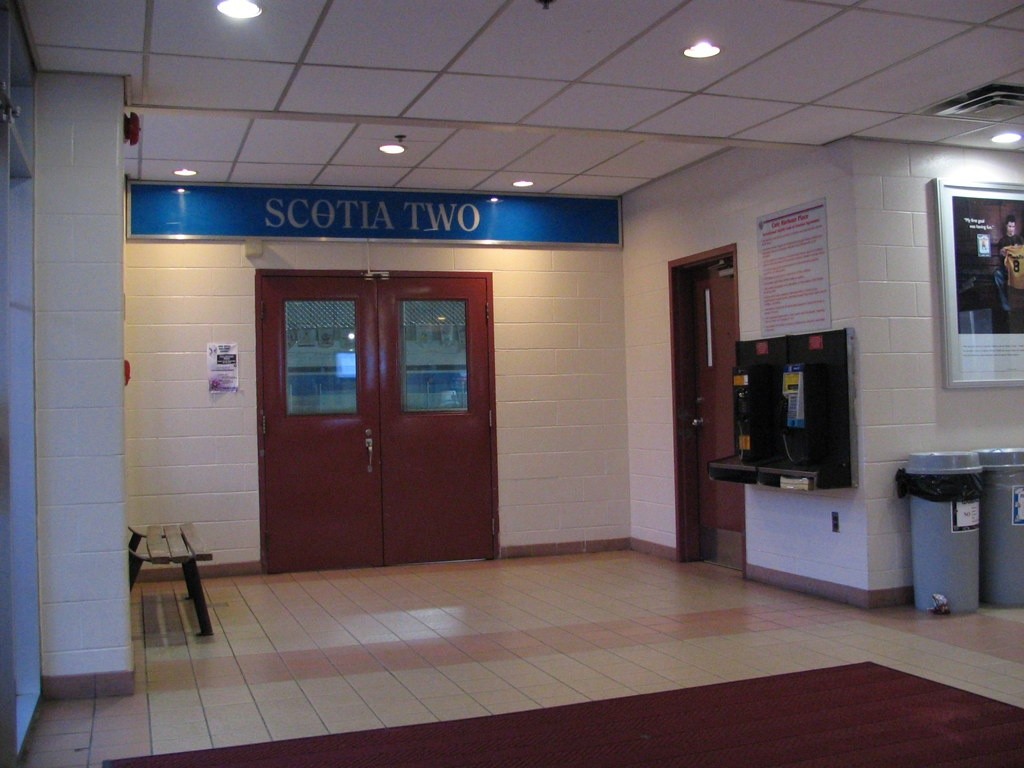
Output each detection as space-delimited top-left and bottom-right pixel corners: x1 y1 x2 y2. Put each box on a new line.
831 512 839 533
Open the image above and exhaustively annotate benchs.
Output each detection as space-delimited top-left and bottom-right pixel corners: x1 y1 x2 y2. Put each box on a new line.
127 521 216 638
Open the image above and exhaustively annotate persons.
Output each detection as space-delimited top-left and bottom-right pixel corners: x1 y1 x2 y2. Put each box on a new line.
992 216 1024 317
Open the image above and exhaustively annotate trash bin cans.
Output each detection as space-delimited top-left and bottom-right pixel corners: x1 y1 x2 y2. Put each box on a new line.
974 448 1024 607
902 452 984 615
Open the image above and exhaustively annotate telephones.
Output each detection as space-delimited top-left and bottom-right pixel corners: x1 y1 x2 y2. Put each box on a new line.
776 359 809 436
732 363 764 435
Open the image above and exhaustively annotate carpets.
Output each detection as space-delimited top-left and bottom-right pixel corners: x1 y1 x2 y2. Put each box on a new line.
100 659 1024 768
